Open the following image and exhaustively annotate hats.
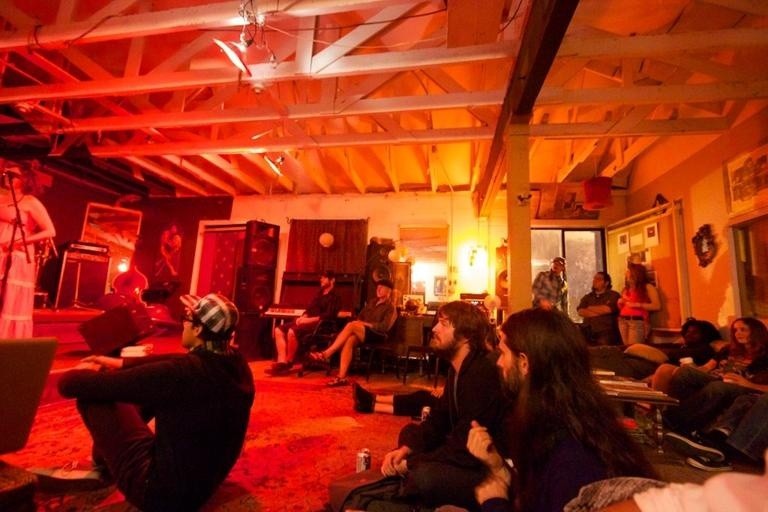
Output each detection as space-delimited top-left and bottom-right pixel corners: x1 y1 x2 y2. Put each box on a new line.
377 279 393 289
179 293 239 334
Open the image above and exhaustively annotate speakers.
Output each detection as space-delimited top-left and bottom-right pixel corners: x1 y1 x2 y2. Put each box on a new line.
232 220 282 361
79 301 155 357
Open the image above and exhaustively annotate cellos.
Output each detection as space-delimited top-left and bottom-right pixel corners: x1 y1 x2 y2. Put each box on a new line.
110 256 149 310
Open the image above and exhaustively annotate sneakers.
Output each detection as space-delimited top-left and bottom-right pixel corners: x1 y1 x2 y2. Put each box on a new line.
327 376 348 386
308 352 331 363
665 432 734 471
25 460 104 487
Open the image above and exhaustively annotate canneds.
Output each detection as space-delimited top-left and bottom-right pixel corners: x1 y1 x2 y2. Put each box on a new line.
357 448 371 472
422 407 430 421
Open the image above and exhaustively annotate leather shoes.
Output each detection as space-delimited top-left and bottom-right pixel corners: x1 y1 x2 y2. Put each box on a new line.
352 383 376 413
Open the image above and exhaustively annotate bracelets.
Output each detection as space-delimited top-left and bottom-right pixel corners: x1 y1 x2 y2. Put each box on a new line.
639 303 642 309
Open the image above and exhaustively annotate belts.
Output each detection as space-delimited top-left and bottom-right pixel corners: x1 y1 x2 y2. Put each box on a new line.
624 316 643 320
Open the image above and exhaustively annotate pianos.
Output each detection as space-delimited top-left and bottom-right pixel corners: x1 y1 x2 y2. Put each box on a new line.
265 303 353 317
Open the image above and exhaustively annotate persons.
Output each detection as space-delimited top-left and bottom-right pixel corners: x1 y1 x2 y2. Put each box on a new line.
530 255 569 317
626 314 722 419
333 299 510 512
576 271 620 346
307 279 399 386
352 330 506 419
263 270 341 375
54 289 256 511
0 157 56 342
433 306 668 512
663 336 768 472
657 316 767 438
614 261 661 347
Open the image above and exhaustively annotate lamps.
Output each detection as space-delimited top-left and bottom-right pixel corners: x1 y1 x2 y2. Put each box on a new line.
582 151 613 211
262 154 285 177
212 2 258 78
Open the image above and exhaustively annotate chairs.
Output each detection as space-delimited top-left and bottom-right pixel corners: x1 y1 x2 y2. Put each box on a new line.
300 300 451 388
461 293 489 306
1 337 57 511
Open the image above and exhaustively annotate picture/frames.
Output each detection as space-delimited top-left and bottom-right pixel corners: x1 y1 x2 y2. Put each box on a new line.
722 135 768 218
433 275 447 296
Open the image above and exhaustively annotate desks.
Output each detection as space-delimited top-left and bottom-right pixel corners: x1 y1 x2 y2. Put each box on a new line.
591 367 680 453
